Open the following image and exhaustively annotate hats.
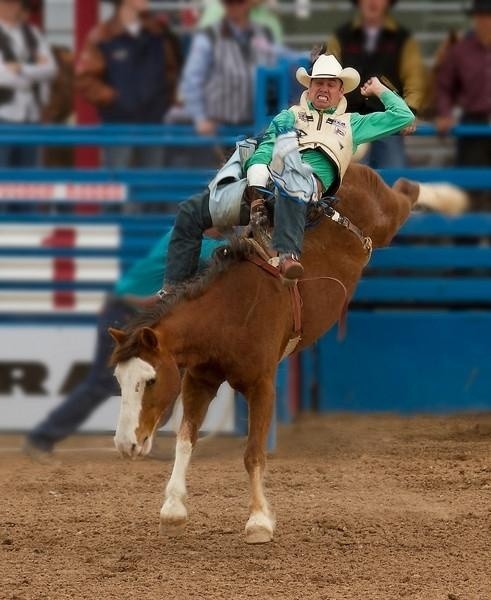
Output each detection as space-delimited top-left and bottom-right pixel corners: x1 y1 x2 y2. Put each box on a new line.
296 54 361 95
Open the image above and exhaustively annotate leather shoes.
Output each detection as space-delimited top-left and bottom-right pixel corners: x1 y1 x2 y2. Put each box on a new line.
274 251 304 288
22 435 60 468
137 285 181 314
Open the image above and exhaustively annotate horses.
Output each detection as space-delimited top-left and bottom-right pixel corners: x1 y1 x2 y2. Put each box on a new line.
105 142 471 545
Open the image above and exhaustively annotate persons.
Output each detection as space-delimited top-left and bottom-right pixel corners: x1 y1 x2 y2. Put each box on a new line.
178 1 273 170
0 0 61 212
24 226 235 466
423 1 491 310
73 0 181 215
325 0 428 167
122 53 416 316
